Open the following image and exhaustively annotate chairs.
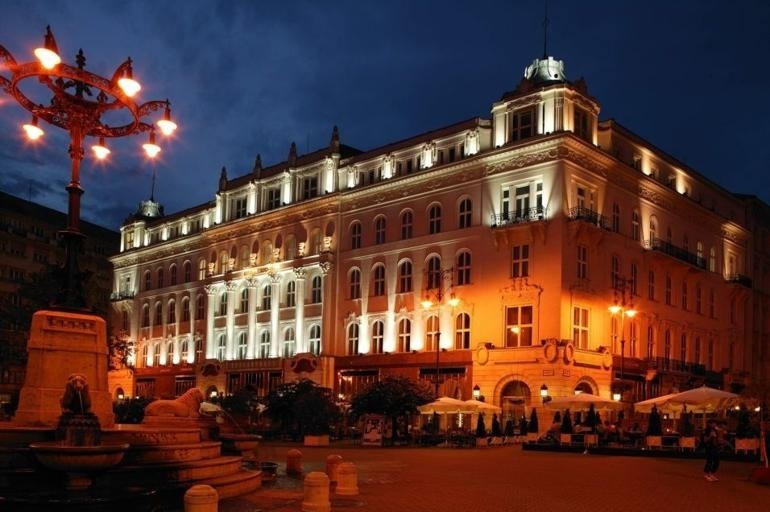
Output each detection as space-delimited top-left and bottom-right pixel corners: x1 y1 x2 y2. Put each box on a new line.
543 424 759 455
450 430 537 449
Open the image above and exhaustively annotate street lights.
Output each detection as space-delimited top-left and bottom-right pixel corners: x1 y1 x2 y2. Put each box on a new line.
609 272 637 441
422 268 459 436
0 24 177 311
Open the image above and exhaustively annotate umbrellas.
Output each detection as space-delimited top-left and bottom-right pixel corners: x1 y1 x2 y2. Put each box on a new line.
634 384 745 429
417 397 502 445
546 393 624 422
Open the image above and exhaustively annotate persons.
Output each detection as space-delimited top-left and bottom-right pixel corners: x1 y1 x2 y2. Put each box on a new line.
702 420 734 482
606 422 641 442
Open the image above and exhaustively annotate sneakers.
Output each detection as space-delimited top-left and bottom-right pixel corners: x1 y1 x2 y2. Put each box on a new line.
704 475 719 481
